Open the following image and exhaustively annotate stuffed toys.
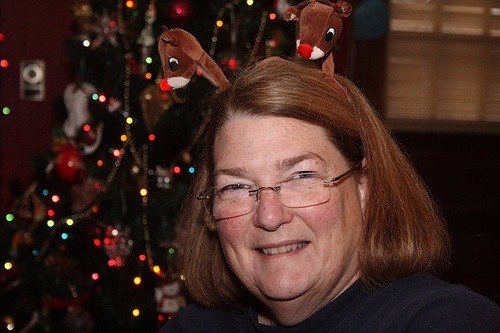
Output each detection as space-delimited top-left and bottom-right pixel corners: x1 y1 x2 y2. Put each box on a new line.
282 1 353 76
158 24 231 90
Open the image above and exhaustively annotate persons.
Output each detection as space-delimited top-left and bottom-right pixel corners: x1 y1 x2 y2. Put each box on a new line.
156 57 500 333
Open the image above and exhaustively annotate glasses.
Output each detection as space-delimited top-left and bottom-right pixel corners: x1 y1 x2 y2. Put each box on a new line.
197 161 362 221
